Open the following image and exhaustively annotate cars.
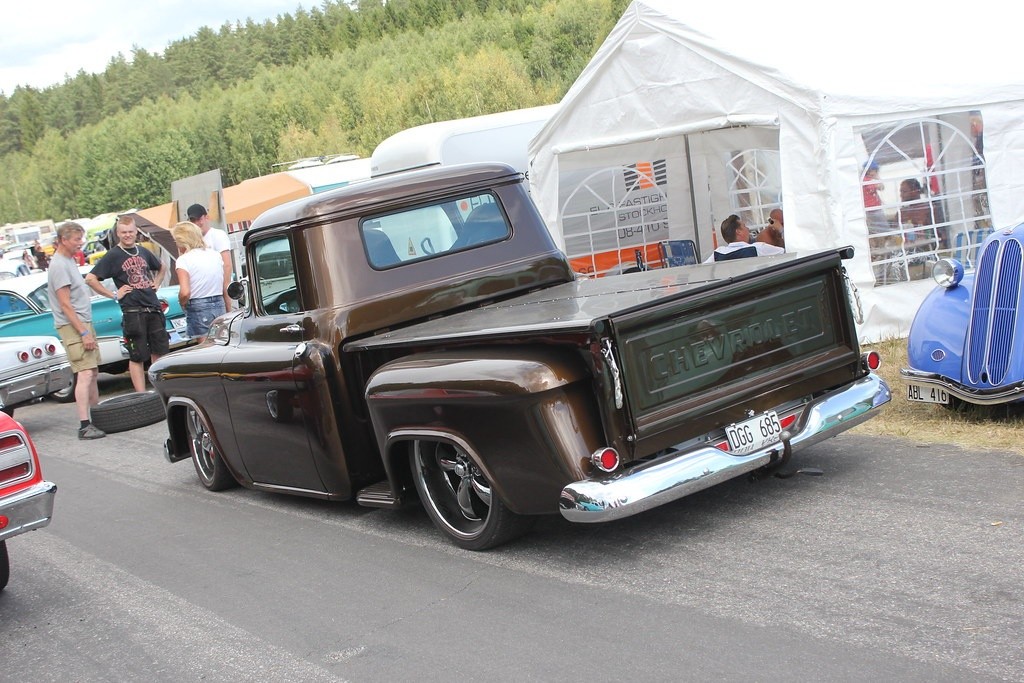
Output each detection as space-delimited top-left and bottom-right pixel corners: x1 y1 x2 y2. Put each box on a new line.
0 211 403 438
900 218 1024 412
0 410 58 589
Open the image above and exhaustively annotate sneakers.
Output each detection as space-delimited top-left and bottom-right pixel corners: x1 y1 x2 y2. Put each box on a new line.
78 424 105 440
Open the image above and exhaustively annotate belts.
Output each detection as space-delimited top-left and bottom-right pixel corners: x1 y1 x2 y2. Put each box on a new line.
122 307 160 313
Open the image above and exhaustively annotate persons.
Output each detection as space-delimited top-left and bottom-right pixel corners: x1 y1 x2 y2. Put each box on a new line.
757 209 784 248
894 178 938 233
33 244 48 272
73 246 85 266
969 115 992 229
85 216 170 391
704 214 784 263
53 240 58 252
22 250 34 270
48 222 106 440
186 205 239 314
170 221 226 344
860 160 890 233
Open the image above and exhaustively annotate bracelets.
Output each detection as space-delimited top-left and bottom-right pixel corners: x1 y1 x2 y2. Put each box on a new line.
779 228 784 234
113 292 119 304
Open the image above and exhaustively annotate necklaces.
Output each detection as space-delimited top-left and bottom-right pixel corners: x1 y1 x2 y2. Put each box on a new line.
117 244 140 257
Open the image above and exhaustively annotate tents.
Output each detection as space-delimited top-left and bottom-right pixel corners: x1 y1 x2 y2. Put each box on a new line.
526 0 1024 345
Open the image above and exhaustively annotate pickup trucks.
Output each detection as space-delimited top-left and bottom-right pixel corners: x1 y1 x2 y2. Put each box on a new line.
148 152 894 552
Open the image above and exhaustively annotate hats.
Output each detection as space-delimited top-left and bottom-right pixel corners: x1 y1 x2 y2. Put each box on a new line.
186 203 207 221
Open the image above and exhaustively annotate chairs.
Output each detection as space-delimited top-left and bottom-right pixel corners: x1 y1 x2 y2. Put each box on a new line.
461 202 508 244
364 230 402 267
952 227 995 269
634 238 700 271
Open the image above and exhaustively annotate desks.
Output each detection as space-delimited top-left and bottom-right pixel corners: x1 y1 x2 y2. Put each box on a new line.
869 238 943 285
